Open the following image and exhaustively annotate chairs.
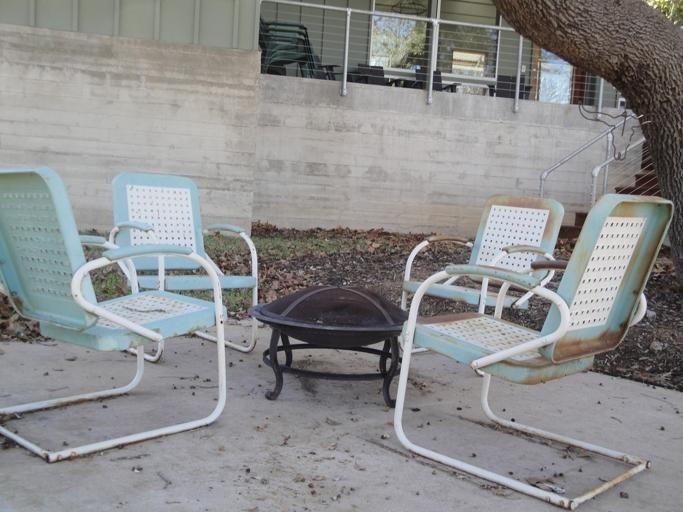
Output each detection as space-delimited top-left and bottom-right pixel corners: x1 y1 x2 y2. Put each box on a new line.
348 62 461 95
393 193 675 512
258 13 316 79
399 193 565 354
0 165 228 463
108 171 257 362
